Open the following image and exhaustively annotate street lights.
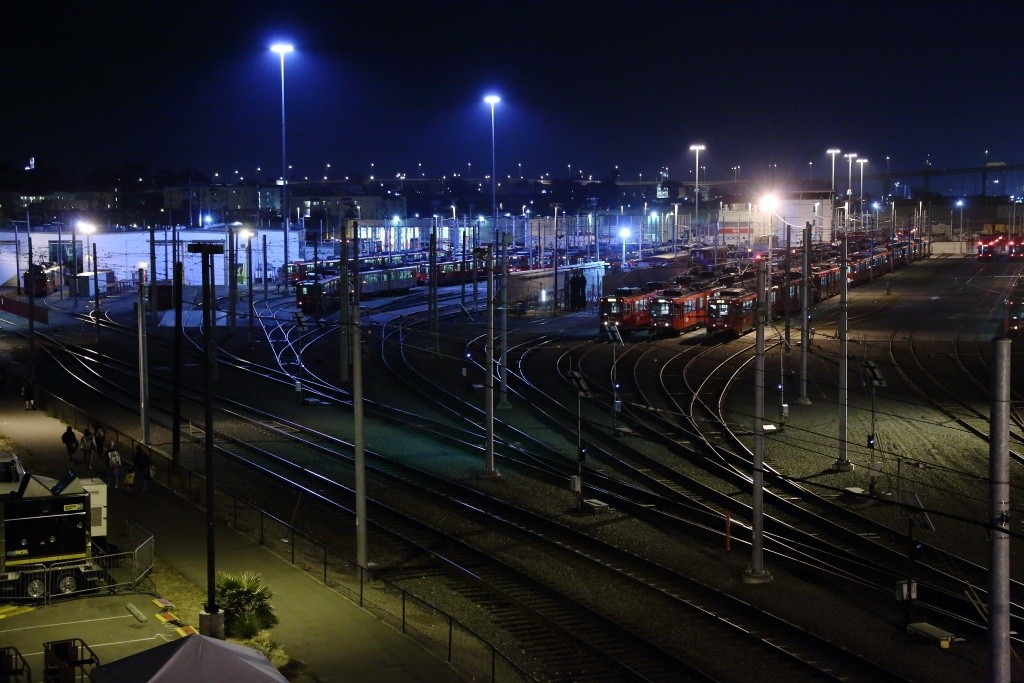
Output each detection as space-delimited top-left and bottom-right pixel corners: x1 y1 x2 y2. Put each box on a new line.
482 93 503 247
214 143 988 239
271 44 294 216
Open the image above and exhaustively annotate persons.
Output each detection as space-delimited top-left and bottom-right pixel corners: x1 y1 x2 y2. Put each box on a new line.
54 421 167 502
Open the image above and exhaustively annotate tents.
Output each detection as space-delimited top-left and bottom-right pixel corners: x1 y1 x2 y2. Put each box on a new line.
88 629 291 683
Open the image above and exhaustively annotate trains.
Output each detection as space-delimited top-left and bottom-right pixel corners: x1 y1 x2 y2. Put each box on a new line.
281 233 1024 339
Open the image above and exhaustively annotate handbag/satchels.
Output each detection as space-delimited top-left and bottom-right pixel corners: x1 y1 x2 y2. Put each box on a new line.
108 450 122 467
149 464 156 479
